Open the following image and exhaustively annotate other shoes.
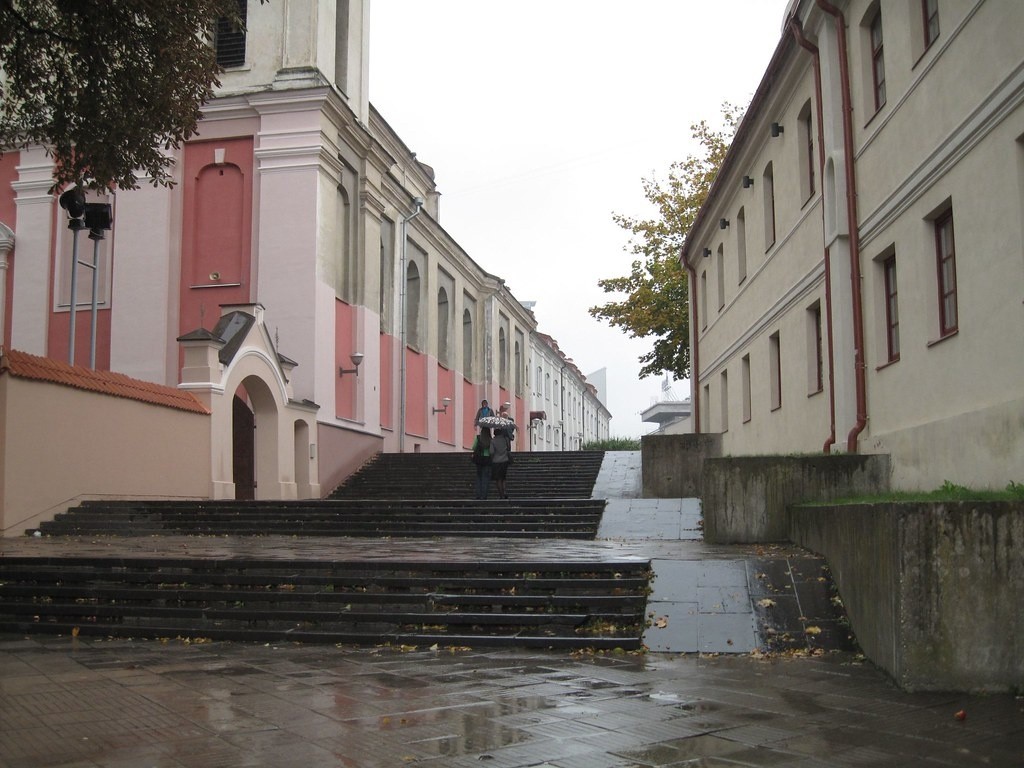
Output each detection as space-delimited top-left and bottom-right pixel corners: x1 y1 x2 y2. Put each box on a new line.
500 494 508 499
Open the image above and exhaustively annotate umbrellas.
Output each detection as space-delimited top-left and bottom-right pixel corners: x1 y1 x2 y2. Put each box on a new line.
474 416 519 429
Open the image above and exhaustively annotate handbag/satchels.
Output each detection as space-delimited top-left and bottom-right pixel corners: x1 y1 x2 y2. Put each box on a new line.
507 451 514 464
472 435 482 465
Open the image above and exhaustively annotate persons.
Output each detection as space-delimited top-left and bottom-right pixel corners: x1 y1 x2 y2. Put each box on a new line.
490 429 511 499
472 427 493 500
475 400 495 427
502 412 514 450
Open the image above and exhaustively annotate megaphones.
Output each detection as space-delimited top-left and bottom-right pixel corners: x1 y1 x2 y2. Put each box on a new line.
210 272 220 280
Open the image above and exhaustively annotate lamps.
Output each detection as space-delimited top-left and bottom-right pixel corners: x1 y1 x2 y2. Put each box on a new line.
495 402 511 417
719 219 729 229
339 353 365 377
432 396 451 414
573 432 582 440
771 123 783 137
743 176 754 188
703 248 711 257
553 420 563 430
527 418 540 430
